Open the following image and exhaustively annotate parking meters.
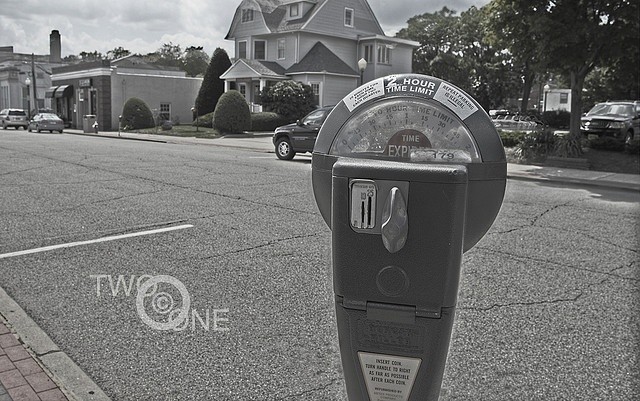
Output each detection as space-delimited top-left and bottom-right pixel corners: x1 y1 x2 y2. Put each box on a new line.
309 72 509 400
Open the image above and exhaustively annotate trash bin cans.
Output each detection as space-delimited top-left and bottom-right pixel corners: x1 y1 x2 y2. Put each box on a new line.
82 115 97 134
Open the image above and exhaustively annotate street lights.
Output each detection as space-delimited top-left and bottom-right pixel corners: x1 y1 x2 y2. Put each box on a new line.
544 84 550 125
25 77 31 121
358 57 367 86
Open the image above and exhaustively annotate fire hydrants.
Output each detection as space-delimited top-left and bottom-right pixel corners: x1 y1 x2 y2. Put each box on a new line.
93 120 99 133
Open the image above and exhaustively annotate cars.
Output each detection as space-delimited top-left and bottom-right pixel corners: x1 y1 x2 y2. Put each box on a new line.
27 112 65 133
580 101 640 146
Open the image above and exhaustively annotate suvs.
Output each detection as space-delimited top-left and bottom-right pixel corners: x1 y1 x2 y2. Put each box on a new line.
0 108 29 129
272 104 334 161
